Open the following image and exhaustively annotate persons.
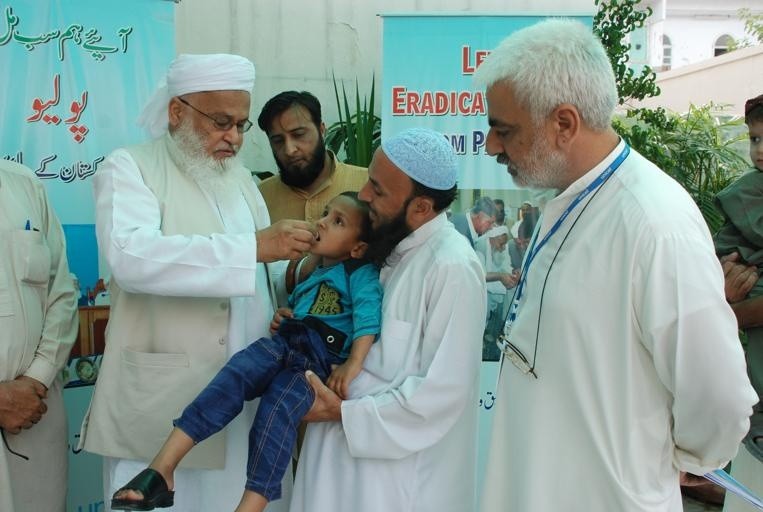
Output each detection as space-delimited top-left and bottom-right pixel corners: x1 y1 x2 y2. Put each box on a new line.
449 198 537 363
110 191 383 510
78 54 320 512
713 94 763 512
257 89 369 259
0 159 81 512
289 128 489 512
472 18 760 512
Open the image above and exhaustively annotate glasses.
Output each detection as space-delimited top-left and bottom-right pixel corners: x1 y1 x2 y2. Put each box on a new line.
177 97 252 133
496 335 533 376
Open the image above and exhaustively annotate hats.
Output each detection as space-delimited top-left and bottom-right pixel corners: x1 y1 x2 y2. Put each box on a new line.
381 128 457 190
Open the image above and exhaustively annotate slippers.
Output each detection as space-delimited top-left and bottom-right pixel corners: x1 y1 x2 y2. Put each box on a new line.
112 469 174 511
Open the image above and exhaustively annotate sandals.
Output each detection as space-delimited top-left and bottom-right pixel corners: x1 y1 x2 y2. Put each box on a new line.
742 415 763 462
